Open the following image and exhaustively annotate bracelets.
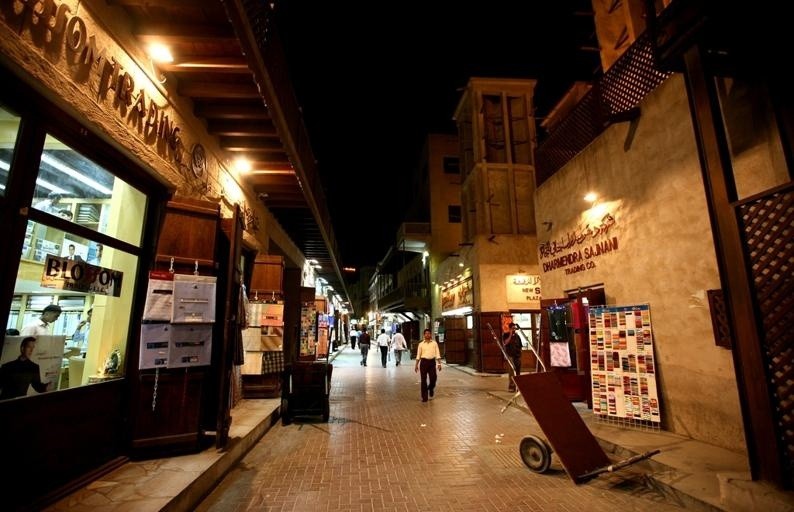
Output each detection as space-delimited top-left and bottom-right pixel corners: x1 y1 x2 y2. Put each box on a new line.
438 364 441 365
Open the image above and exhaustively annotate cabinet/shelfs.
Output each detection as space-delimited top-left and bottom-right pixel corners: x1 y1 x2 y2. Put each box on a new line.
20 197 112 265
6 292 95 367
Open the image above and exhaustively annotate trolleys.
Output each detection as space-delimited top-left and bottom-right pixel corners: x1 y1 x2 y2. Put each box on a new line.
280 327 333 426
487 322 660 485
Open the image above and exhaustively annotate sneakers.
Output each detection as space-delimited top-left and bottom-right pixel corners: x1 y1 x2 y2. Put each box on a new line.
428 386 434 397
421 398 428 402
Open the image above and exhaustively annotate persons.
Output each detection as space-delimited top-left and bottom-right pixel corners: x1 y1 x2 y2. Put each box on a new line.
20 304 63 340
349 328 357 349
34 191 62 214
357 328 370 366
71 309 95 359
501 322 523 392
413 328 443 402
356 328 363 348
376 329 391 368
93 243 103 264
59 210 73 221
0 337 53 401
390 328 408 367
64 244 83 261
